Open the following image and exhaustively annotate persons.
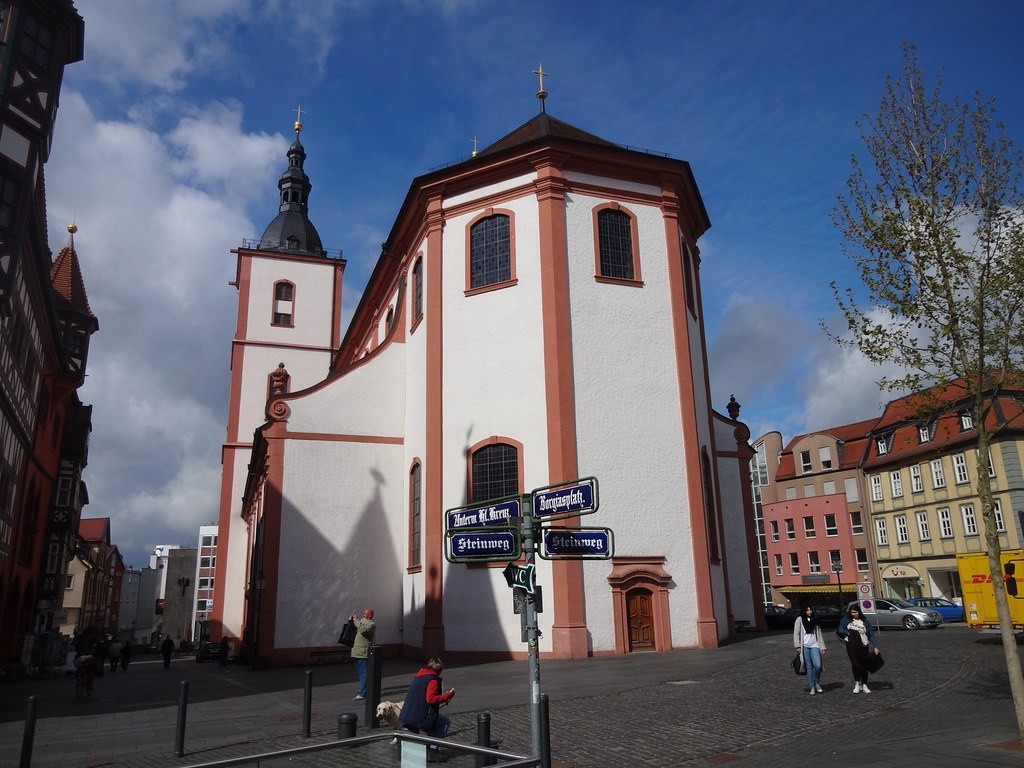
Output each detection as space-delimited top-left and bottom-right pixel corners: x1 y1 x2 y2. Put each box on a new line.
838 605 879 694
348 609 376 701
220 636 229 660
161 634 175 671
793 605 827 695
399 657 455 762
74 625 101 690
109 639 121 672
96 640 105 672
120 641 131 671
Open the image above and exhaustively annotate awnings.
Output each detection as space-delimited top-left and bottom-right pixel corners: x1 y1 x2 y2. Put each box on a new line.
775 584 858 592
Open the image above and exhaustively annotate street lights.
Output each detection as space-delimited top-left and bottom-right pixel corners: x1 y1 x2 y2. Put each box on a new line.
832 551 845 618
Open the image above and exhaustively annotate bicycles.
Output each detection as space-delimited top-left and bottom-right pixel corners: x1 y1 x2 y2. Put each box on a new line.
75 640 105 701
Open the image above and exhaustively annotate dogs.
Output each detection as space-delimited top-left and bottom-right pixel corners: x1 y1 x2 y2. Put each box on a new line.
376 701 405 745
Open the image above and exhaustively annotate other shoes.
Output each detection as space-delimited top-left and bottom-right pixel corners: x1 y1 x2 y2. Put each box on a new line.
810 687 815 694
816 683 823 692
354 695 364 699
427 745 449 762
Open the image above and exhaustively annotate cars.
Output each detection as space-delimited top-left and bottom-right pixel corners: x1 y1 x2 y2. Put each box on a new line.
195 642 221 663
763 606 845 627
907 598 966 623
846 598 944 630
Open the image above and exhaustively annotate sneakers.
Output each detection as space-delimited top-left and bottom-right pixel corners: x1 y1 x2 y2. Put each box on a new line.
853 684 862 693
860 684 871 694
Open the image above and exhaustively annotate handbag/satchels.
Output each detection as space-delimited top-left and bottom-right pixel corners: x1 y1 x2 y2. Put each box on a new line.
338 616 357 648
836 618 850 644
791 652 807 675
867 651 885 674
